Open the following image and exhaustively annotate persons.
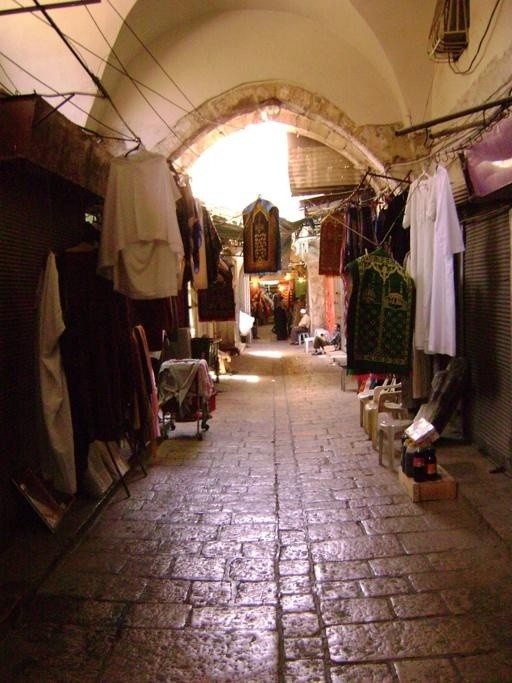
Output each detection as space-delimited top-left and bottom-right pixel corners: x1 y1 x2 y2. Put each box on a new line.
310 322 342 355
289 306 310 345
273 302 289 340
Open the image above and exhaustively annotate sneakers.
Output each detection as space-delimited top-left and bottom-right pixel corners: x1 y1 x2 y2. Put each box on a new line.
290 341 326 355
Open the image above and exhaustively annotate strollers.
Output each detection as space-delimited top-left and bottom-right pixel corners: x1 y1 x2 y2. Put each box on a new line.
157 359 215 440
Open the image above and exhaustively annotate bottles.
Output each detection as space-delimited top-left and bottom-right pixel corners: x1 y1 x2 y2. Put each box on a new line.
401 435 437 482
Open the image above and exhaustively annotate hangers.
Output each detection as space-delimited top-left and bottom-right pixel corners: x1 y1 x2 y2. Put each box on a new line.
417 168 433 185
432 164 446 174
125 137 153 158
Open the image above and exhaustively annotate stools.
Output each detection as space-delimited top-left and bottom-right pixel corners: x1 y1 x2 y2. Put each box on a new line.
378 417 413 473
298 332 309 345
304 337 315 354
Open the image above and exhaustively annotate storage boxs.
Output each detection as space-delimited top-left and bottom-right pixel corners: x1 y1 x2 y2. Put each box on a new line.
399 466 457 501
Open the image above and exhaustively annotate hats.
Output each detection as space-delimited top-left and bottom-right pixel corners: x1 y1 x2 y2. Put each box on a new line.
300 309 307 313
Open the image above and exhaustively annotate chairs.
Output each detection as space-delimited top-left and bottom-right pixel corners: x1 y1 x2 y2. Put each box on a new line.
363 385 404 448
356 371 398 428
